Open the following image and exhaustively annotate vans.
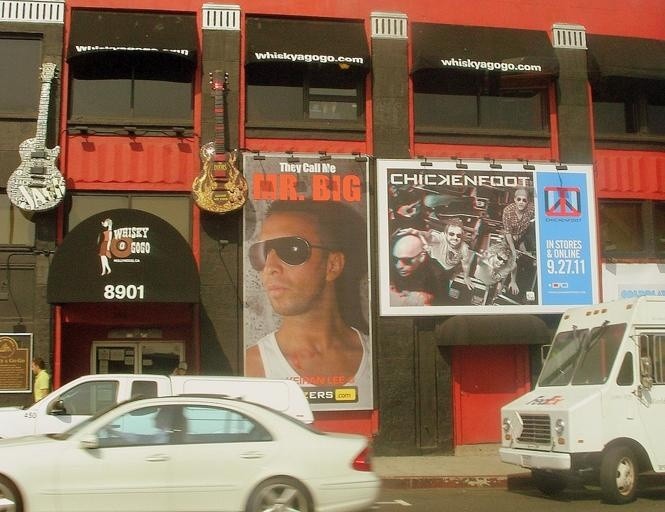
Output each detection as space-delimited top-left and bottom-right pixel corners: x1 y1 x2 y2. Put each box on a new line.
0 367 317 441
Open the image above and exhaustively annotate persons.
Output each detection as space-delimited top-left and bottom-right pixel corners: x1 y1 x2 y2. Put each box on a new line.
390 233 448 306
469 240 521 305
500 189 536 262
398 220 476 292
29 357 49 402
244 199 373 411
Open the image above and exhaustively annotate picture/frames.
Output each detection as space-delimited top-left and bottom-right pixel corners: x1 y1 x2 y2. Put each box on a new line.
0 332 33 393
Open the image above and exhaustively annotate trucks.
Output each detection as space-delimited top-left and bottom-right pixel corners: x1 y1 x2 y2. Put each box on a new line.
498 296 665 504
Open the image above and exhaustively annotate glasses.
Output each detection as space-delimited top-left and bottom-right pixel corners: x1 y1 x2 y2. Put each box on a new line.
248 237 329 272
392 252 422 265
496 253 507 264
517 197 528 202
448 231 462 238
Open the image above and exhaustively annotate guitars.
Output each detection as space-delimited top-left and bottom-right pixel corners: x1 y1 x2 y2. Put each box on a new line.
192 70 249 216
6 63 67 213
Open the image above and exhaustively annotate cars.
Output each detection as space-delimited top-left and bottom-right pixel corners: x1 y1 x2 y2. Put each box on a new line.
0 395 383 512
